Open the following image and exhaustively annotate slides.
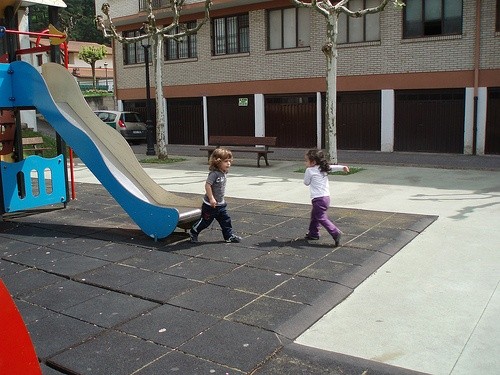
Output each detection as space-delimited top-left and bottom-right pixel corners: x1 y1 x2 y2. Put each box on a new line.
11 60 210 239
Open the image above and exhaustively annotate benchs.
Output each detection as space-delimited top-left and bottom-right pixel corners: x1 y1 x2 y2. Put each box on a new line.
22 137 52 157
200 136 277 168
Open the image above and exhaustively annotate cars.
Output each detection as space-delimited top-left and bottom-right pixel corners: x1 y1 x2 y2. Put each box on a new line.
92 110 146 144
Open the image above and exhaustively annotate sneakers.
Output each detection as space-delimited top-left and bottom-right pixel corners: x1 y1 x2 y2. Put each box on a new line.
305 233 320 239
333 232 343 246
187 229 199 243
224 234 242 243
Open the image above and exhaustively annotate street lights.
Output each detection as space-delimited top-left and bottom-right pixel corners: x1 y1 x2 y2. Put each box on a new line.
139 22 156 155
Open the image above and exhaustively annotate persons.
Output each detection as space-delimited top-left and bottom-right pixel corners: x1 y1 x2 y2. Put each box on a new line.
303 149 348 242
190 148 242 243
